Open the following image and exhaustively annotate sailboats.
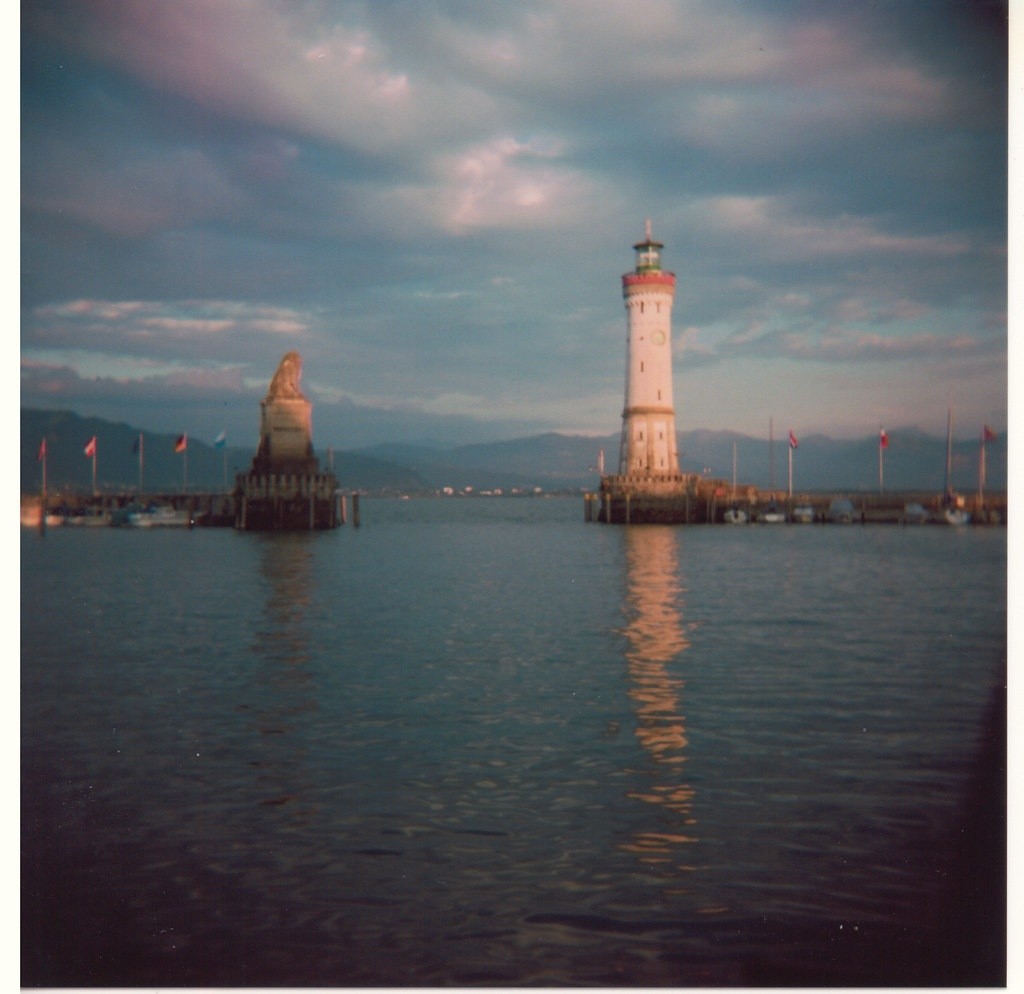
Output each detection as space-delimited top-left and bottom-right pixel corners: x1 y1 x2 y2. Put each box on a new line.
723 440 748 525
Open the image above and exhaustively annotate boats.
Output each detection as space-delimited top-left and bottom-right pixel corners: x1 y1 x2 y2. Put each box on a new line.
47 499 193 528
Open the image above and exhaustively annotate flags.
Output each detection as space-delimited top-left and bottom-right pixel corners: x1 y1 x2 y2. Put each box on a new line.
175 432 186 454
131 436 140 454
984 423 997 443
84 434 94 457
215 428 225 449
39 440 45 461
879 427 888 449
789 429 799 449
